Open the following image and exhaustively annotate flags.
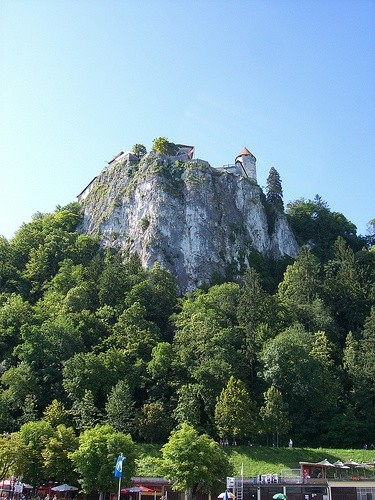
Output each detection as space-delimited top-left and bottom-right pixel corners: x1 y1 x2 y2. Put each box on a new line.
115 456 122 477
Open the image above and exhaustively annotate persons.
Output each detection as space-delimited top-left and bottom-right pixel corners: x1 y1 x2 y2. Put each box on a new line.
289 439 292 448
0 490 57 500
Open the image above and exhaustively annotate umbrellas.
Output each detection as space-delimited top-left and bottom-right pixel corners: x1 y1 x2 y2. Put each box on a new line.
272 494 287 500
14 481 34 488
300 459 334 479
217 492 236 499
334 459 375 479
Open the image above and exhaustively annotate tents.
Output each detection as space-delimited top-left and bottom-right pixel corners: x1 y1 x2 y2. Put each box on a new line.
51 484 78 491
121 485 156 500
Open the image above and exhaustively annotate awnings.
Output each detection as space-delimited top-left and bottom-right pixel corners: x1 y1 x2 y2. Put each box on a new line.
328 480 375 500
